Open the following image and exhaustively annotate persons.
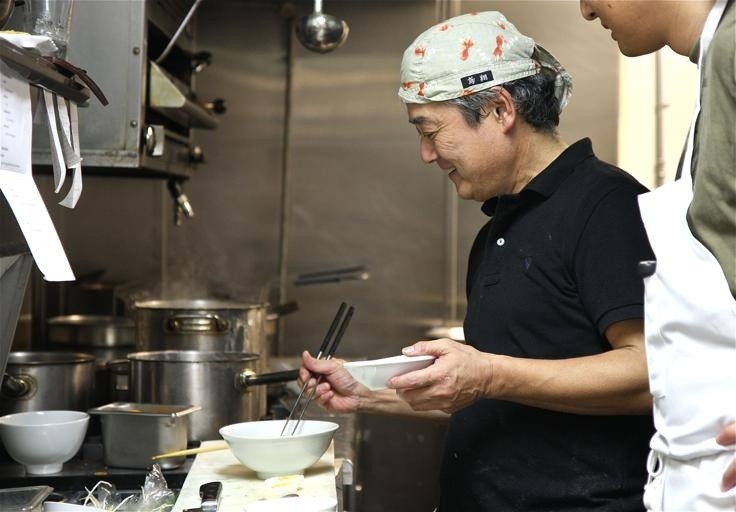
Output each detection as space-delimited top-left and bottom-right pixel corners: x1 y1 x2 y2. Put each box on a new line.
296 9 653 511
579 0 736 512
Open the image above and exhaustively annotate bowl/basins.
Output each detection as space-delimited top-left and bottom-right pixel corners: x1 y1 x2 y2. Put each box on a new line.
0 409 90 476
343 349 434 391
215 416 340 481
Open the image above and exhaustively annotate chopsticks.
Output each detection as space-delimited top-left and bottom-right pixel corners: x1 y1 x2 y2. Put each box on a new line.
280 300 355 435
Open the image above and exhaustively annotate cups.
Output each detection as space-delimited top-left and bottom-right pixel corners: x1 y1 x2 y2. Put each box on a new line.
23 0 74 66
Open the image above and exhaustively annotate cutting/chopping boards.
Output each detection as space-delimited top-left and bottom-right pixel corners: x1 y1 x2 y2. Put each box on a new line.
169 435 338 512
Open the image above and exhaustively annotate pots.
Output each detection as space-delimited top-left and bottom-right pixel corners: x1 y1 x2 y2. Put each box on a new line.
104 358 131 404
45 313 134 362
131 299 270 421
126 348 301 445
1 350 98 411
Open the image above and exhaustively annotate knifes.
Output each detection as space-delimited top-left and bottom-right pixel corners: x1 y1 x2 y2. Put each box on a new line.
181 480 222 512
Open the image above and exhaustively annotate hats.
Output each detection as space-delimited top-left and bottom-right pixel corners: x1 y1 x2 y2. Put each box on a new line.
398 11 573 115
